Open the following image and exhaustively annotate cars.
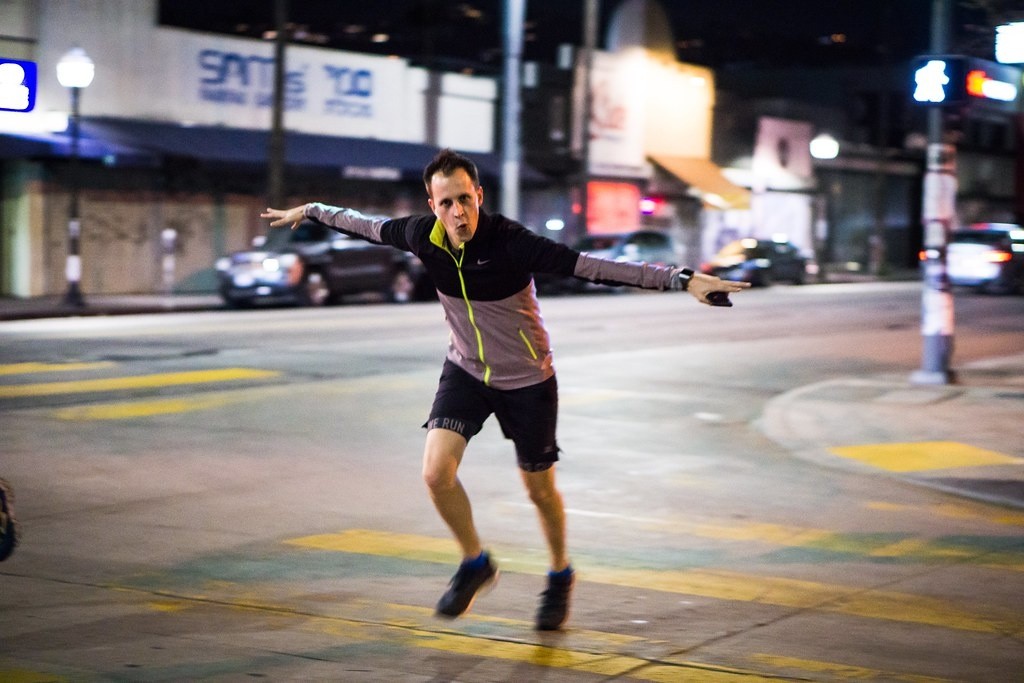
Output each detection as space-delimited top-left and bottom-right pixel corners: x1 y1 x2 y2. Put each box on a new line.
943 221 1024 297
548 230 690 295
697 237 810 290
211 217 440 310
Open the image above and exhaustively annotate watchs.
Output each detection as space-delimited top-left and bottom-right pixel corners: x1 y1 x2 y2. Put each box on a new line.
679 268 696 291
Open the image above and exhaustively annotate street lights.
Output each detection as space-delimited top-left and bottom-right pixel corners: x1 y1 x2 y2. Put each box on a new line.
55 46 95 317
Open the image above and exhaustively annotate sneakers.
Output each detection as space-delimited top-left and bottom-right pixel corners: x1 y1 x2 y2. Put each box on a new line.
531 563 577 632
432 551 503 623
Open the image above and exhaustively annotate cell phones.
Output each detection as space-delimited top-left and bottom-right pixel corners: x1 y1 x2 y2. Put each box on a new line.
707 290 733 308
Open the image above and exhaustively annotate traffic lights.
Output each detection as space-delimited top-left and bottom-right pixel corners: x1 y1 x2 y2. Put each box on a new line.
912 50 1024 124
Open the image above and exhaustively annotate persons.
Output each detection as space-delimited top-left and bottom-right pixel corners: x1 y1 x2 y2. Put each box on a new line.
260 149 750 629
